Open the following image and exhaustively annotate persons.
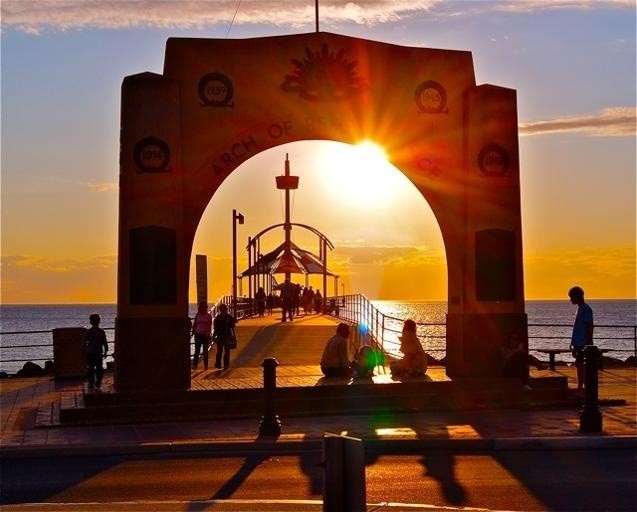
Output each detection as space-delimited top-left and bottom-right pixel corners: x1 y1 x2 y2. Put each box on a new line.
320 322 353 378
192 301 213 371
252 277 322 322
390 319 427 376
567 284 595 398
498 330 549 391
213 304 236 370
351 342 374 378
79 313 109 389
188 316 194 341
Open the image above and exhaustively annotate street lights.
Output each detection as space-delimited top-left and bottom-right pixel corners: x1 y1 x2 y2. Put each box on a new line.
233 208 264 320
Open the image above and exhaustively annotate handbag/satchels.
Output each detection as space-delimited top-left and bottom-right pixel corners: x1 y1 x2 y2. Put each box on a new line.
227 327 237 350
